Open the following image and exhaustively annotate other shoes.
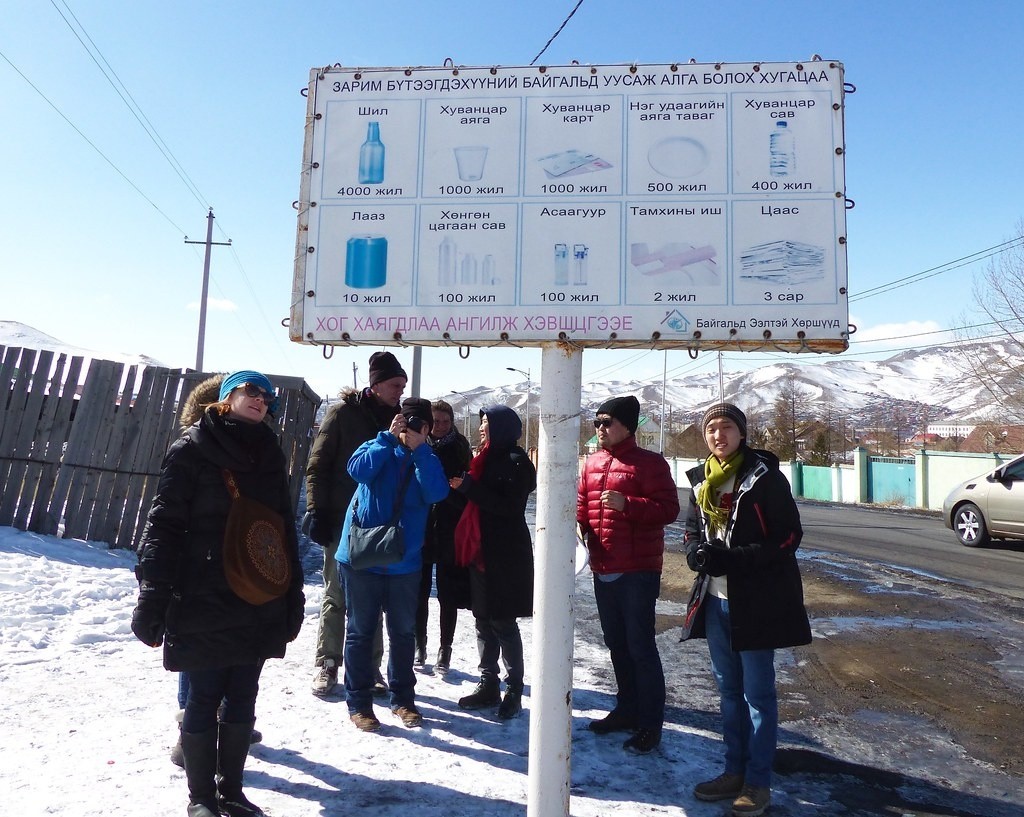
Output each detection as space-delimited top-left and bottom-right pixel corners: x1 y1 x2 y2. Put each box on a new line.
414 647 426 665
437 646 452 671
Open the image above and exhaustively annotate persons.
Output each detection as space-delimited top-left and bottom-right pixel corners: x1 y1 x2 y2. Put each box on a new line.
681 402 811 817
333 397 451 732
575 394 681 755
130 370 305 817
448 404 537 719
413 398 475 673
305 350 407 695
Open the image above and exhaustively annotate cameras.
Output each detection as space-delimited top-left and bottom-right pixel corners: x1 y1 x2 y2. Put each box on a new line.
693 537 725 565
397 416 427 435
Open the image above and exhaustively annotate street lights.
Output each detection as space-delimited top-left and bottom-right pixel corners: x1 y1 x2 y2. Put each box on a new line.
506 367 531 454
451 390 470 444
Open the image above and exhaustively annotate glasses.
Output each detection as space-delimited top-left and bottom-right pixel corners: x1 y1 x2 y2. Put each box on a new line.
593 419 618 429
238 381 276 406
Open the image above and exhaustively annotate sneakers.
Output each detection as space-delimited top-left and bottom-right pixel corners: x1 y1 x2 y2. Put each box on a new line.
497 682 524 719
694 771 740 800
730 781 771 817
393 705 423 727
623 728 662 753
310 658 339 695
351 710 382 732
371 668 388 697
458 680 502 711
588 710 638 736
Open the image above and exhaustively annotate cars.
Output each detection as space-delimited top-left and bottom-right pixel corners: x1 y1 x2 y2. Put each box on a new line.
943 452 1024 548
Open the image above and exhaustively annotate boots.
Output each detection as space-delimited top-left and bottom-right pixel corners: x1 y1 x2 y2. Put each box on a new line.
250 730 262 745
171 708 186 766
218 715 264 817
180 727 221 817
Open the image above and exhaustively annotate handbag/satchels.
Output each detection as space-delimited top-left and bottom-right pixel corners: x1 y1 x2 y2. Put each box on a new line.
348 523 404 570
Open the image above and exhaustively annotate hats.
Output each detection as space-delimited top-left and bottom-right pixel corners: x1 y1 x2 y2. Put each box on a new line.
702 403 747 449
220 371 274 409
400 396 434 429
596 396 640 434
369 352 407 390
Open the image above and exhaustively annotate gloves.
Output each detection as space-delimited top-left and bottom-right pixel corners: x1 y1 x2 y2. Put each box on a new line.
686 544 728 577
131 575 170 648
308 517 333 549
286 590 306 643
